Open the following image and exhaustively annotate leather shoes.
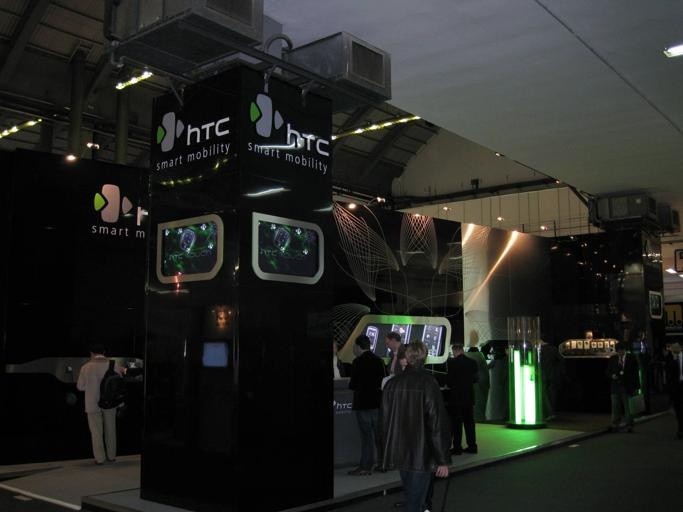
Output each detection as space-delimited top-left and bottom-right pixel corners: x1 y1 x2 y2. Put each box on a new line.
348 467 371 475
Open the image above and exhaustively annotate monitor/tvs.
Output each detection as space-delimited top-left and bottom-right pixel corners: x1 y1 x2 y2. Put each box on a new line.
649 291 663 320
252 212 325 284
200 340 228 368
156 214 223 282
333 377 350 389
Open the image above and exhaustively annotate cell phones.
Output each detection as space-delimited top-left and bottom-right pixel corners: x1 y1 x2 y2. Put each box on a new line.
366 325 380 353
386 324 411 356
421 326 442 357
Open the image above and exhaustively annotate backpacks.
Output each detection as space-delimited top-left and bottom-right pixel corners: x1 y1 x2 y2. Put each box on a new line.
98 359 126 409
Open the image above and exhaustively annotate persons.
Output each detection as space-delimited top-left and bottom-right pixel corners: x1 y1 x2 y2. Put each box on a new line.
666 340 683 440
606 342 641 433
348 332 509 512
539 336 564 421
76 347 129 464
